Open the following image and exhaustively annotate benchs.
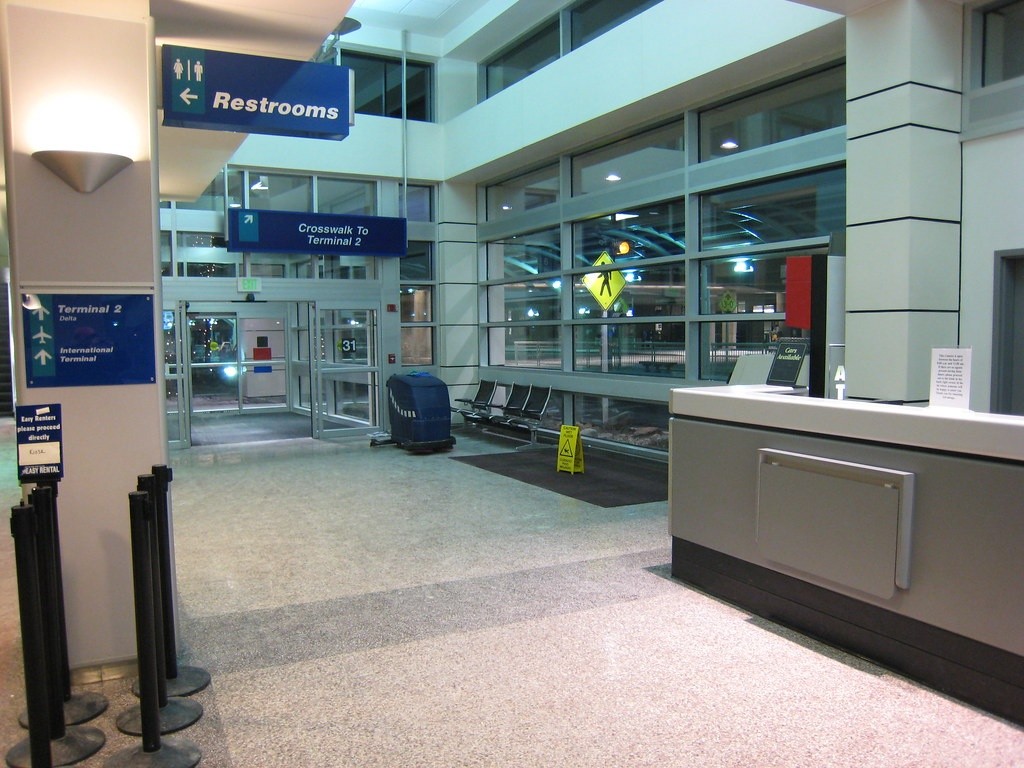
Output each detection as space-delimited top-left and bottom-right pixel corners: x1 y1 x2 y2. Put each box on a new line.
450 378 552 450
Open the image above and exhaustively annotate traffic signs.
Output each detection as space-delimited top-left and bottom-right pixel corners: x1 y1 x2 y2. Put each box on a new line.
162 44 350 141
228 208 409 258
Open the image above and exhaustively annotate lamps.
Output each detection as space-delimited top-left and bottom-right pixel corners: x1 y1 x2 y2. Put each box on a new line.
31 151 135 194
